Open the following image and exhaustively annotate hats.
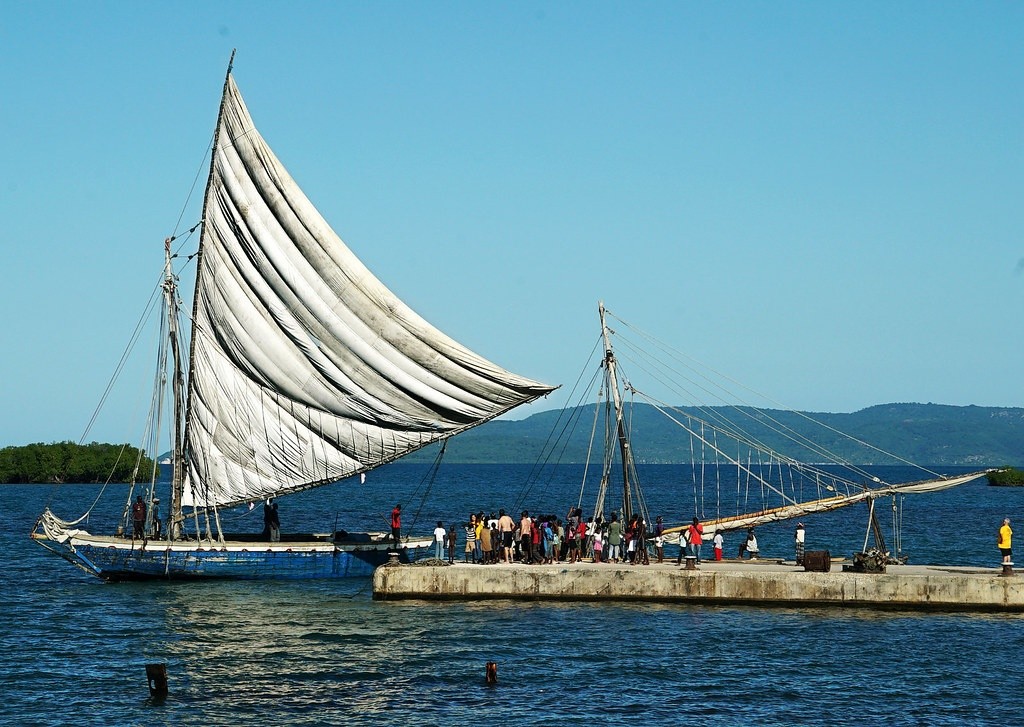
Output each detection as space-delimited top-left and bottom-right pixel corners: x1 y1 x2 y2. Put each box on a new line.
152 498 159 502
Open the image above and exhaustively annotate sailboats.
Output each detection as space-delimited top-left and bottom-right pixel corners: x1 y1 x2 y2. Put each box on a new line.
18 45 569 583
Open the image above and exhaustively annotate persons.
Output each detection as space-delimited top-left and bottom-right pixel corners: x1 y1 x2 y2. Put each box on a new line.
152 498 161 539
464 509 649 565
132 496 146 540
675 529 688 566
520 511 531 563
447 526 456 563
392 504 402 539
688 517 703 565
998 518 1013 562
714 529 724 561
261 498 281 542
736 528 758 559
433 521 446 560
566 505 583 563
655 516 664 563
794 522 806 566
605 514 622 563
